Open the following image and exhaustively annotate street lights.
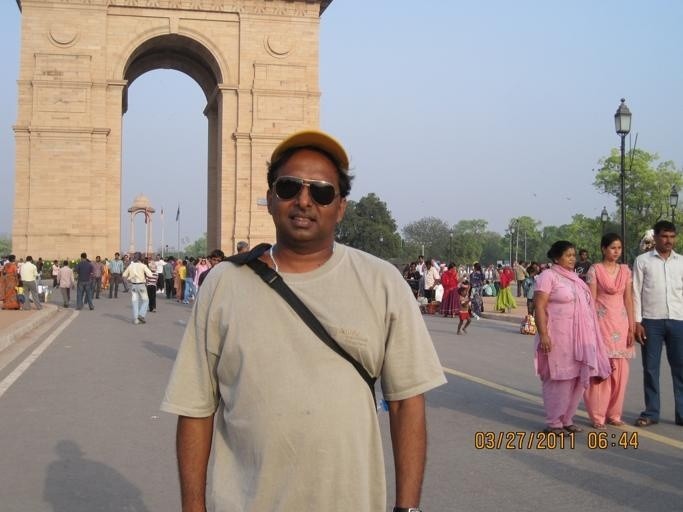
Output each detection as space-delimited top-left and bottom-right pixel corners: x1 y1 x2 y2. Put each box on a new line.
669 183 680 243
378 232 383 258
510 226 516 268
601 208 609 236
613 95 633 263
448 228 455 264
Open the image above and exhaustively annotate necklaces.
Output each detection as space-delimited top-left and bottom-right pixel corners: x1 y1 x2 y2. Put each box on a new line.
268 241 282 275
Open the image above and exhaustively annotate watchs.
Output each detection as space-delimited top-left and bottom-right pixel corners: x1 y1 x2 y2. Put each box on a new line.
393 507 422 512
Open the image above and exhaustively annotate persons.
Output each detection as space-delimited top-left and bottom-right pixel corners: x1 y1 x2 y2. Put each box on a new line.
632 220 683 426
585 234 636 431
533 240 613 434
158 129 450 512
453 280 474 333
1 249 596 326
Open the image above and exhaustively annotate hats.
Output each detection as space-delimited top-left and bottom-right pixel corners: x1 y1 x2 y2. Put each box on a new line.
270 130 349 168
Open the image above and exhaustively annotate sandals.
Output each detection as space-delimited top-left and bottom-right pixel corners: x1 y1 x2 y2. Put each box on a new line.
636 417 657 426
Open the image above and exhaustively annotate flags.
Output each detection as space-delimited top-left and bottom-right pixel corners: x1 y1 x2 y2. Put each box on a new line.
160 206 166 219
175 204 180 219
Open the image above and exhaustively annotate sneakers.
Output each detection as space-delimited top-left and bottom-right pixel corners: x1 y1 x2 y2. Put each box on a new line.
543 425 582 435
593 420 625 429
137 315 145 323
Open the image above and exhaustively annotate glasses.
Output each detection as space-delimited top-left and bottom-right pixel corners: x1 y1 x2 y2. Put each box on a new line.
269 175 340 206
